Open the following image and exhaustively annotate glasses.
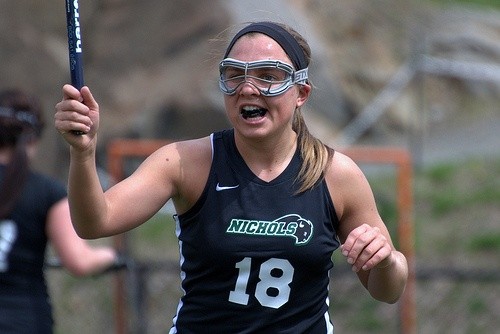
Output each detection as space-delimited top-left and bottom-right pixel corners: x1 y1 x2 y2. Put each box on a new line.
219 58 309 96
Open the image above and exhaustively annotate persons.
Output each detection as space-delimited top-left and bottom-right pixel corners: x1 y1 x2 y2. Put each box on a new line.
0 88 119 334
54 20 409 334
